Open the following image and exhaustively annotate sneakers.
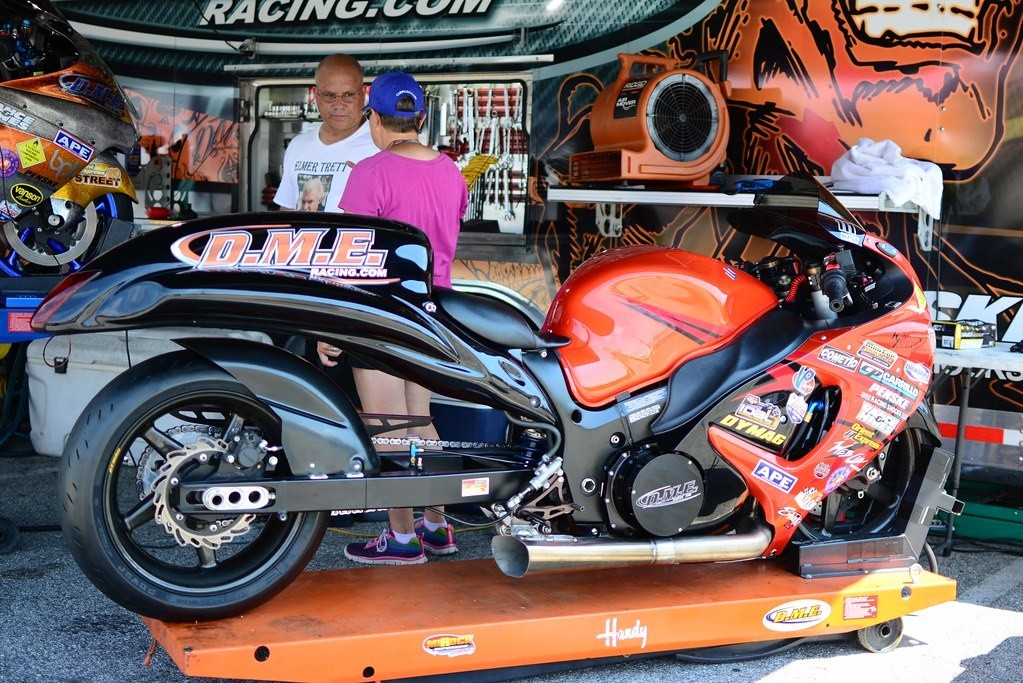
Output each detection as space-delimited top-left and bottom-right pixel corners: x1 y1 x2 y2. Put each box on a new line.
413 518 458 555
344 521 428 565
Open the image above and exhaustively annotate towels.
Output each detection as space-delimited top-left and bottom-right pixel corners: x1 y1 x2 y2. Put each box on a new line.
829 136 944 222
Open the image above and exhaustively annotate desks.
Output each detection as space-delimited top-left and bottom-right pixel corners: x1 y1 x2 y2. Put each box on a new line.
917 338 1023 561
548 184 936 253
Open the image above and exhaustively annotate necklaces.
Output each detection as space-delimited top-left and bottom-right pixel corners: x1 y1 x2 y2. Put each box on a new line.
387 139 420 149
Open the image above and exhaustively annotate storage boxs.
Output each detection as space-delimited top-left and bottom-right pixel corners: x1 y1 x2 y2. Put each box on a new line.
23 328 272 467
932 319 996 351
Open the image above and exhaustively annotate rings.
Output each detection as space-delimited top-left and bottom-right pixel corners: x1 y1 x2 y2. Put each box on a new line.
333 346 336 351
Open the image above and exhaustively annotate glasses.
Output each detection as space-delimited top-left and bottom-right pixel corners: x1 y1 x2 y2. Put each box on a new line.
315 88 363 103
364 108 373 121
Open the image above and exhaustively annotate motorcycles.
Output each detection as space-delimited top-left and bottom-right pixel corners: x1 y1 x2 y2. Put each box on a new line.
0 0 141 450
27 153 968 624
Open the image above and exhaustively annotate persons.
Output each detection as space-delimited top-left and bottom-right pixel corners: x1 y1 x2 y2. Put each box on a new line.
317 73 469 565
272 53 382 411
301 179 326 211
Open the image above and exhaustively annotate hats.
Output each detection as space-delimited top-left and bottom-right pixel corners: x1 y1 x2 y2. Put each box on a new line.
360 71 424 119
